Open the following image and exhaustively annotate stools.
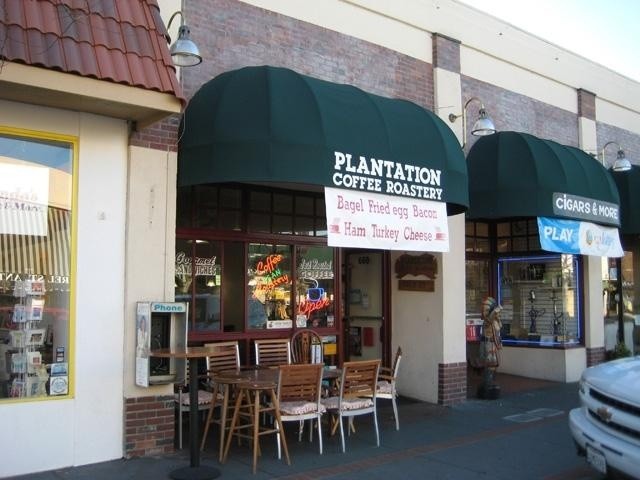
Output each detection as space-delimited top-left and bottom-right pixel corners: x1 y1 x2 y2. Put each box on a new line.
221 380 294 467
200 375 263 463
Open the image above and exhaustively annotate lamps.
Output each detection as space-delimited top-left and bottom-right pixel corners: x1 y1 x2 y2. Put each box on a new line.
163 9 204 69
449 97 496 136
588 139 634 173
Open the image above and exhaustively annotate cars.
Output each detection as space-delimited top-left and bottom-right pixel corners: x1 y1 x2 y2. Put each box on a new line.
568 356 640 480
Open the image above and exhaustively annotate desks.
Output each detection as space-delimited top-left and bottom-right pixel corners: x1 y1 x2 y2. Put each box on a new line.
240 366 345 442
148 346 238 478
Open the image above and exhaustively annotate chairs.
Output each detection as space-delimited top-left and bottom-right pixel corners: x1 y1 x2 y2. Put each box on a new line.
325 358 383 454
253 337 293 367
267 364 328 457
331 345 404 433
202 339 256 434
290 329 325 364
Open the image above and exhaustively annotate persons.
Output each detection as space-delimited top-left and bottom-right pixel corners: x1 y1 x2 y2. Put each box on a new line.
481 296 503 390
